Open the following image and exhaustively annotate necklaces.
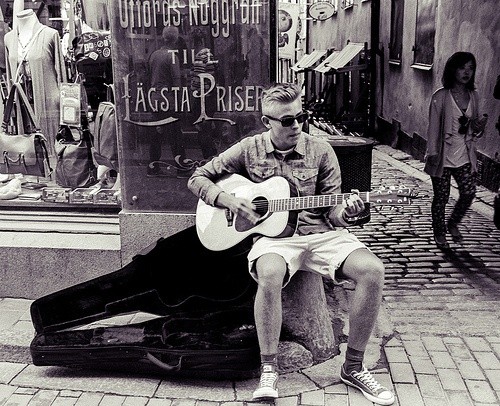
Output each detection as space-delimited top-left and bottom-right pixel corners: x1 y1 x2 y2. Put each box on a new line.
457 89 466 98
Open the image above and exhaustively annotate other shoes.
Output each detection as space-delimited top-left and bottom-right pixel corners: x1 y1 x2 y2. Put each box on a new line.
175 169 195 178
147 165 169 177
448 224 463 242
433 234 450 249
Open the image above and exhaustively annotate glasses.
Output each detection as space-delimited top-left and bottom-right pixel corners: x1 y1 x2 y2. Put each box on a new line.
458 114 468 135
265 108 310 127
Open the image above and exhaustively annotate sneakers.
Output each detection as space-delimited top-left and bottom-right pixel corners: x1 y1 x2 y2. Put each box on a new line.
252 364 280 398
339 362 395 405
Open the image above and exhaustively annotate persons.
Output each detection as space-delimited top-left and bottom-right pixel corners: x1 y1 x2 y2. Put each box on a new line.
247 35 270 85
146 26 194 178
4 8 67 133
187 82 395 406
423 52 486 248
493 75 500 204
189 49 221 159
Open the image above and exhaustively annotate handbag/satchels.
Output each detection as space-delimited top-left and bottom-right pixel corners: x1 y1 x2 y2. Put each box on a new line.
0 82 54 177
91 83 119 172
72 20 112 73
52 126 99 188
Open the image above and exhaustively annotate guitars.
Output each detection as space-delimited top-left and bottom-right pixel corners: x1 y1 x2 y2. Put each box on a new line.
193 172 419 253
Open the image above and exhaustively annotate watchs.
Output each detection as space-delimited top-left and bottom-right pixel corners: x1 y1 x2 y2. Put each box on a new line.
343 211 364 221
